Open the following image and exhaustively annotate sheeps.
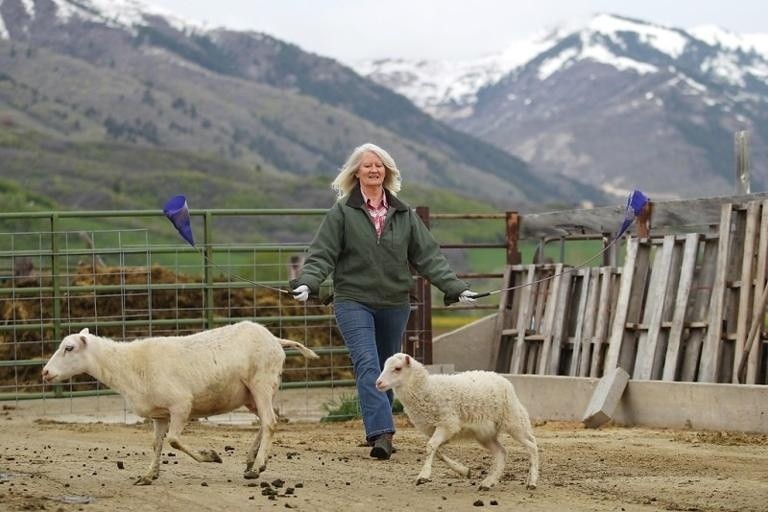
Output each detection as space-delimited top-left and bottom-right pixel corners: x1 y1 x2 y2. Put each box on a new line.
373 350 540 492
39 319 321 488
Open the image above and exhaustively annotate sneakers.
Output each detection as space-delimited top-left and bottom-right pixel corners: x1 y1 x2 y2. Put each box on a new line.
367 434 395 460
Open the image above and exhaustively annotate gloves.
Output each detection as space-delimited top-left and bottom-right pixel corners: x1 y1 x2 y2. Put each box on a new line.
458 289 478 302
292 285 312 301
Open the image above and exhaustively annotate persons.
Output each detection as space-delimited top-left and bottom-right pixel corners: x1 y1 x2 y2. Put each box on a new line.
287 144 477 460
